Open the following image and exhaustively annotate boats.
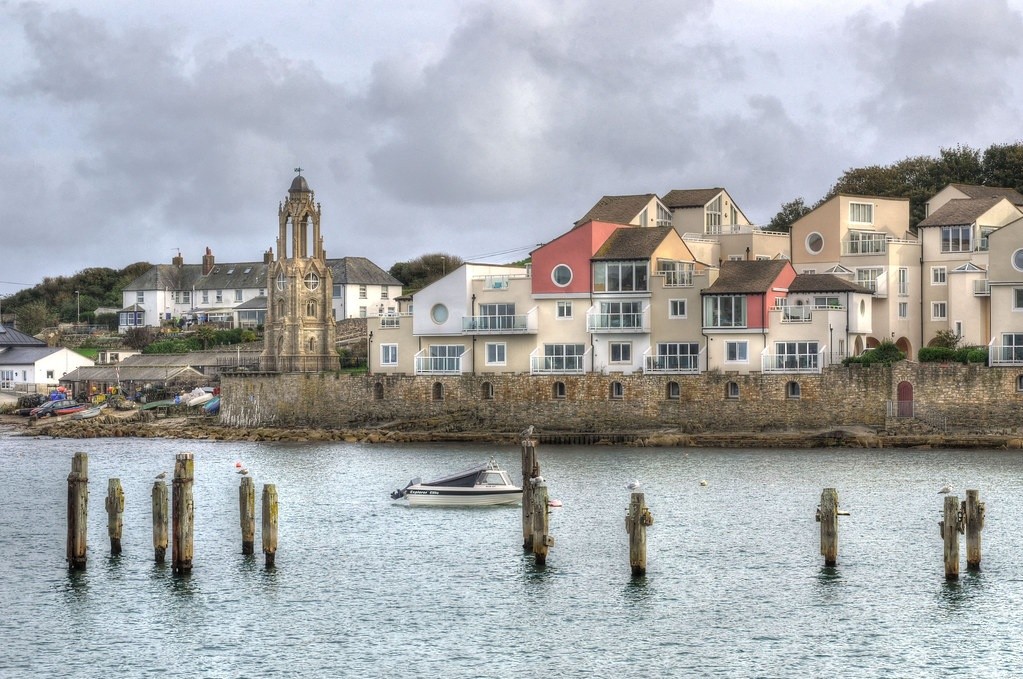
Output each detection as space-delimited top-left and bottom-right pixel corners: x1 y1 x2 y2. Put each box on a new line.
72 407 100 419
390 456 523 505
55 403 85 415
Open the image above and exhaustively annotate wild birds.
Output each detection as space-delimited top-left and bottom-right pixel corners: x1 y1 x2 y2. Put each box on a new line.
937 484 952 496
527 476 546 485
519 425 535 440
624 477 639 492
234 469 249 476
153 471 169 479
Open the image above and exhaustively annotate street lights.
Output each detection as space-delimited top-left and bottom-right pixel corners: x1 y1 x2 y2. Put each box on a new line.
75 291 79 325
441 257 445 276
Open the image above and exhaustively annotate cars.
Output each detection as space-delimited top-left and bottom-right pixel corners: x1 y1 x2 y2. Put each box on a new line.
30 399 79 417
